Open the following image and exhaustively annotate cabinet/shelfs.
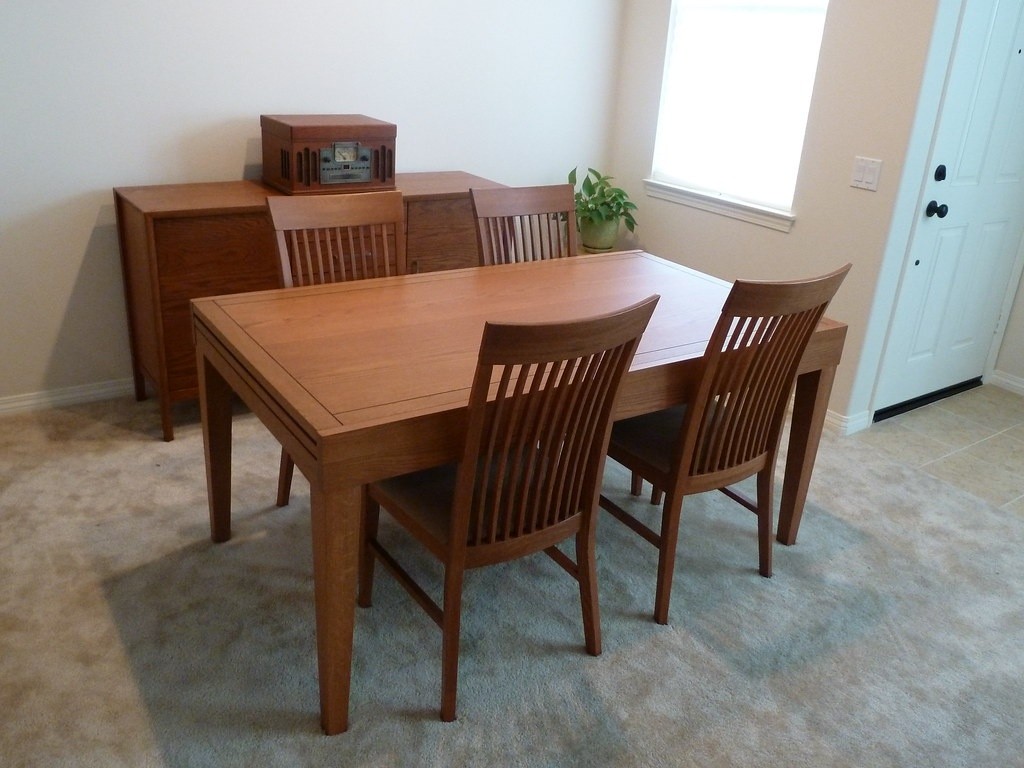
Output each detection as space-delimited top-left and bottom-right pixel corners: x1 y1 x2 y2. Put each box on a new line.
111 172 507 443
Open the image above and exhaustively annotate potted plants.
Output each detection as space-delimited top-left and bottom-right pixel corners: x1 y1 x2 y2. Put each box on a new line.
553 164 638 254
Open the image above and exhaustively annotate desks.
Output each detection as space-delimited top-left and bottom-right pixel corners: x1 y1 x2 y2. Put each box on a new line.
186 246 849 736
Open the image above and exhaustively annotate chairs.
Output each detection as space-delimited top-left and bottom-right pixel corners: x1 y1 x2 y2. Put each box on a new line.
265 190 406 507
467 184 576 267
590 263 852 624
351 295 660 722
